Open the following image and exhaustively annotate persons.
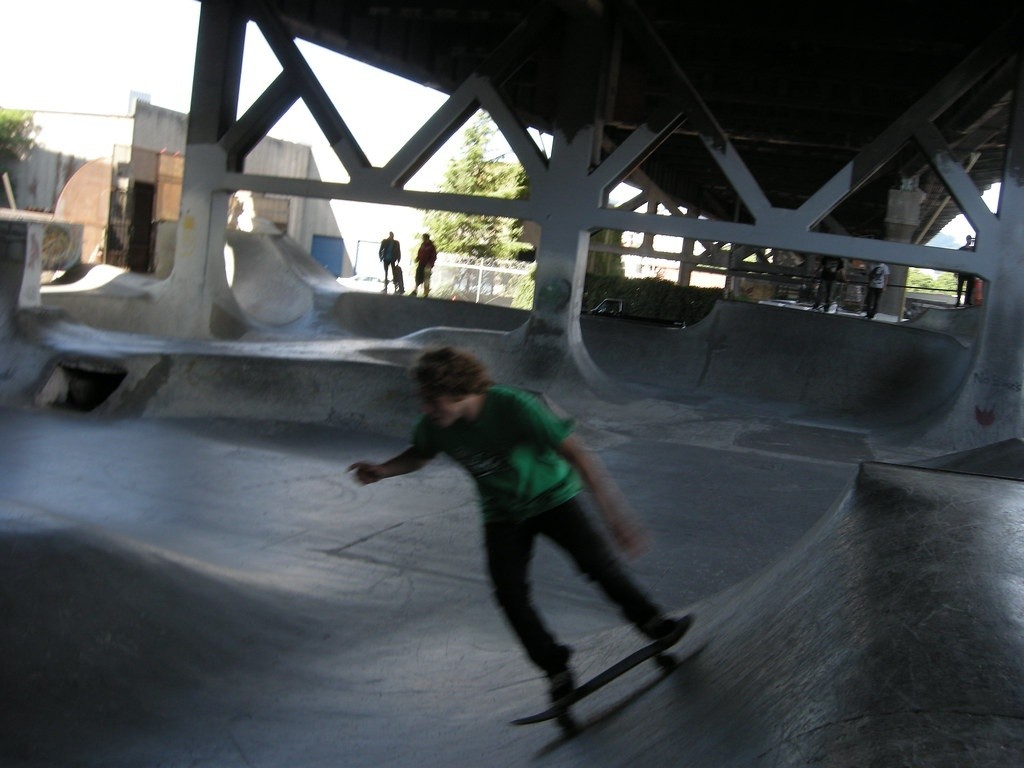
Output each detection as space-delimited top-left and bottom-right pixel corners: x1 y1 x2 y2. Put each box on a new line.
379 232 402 294
410 234 437 297
810 257 848 312
866 263 891 320
343 346 695 701
954 235 975 307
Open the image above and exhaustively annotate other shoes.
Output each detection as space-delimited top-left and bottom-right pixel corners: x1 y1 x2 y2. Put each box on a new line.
645 618 678 638
549 672 573 697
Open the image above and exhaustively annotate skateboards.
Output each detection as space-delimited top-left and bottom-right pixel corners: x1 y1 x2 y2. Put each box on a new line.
508 611 699 727
393 266 405 295
826 281 842 314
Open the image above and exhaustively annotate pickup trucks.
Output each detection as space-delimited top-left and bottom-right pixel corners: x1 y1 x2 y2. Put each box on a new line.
590 298 685 329
